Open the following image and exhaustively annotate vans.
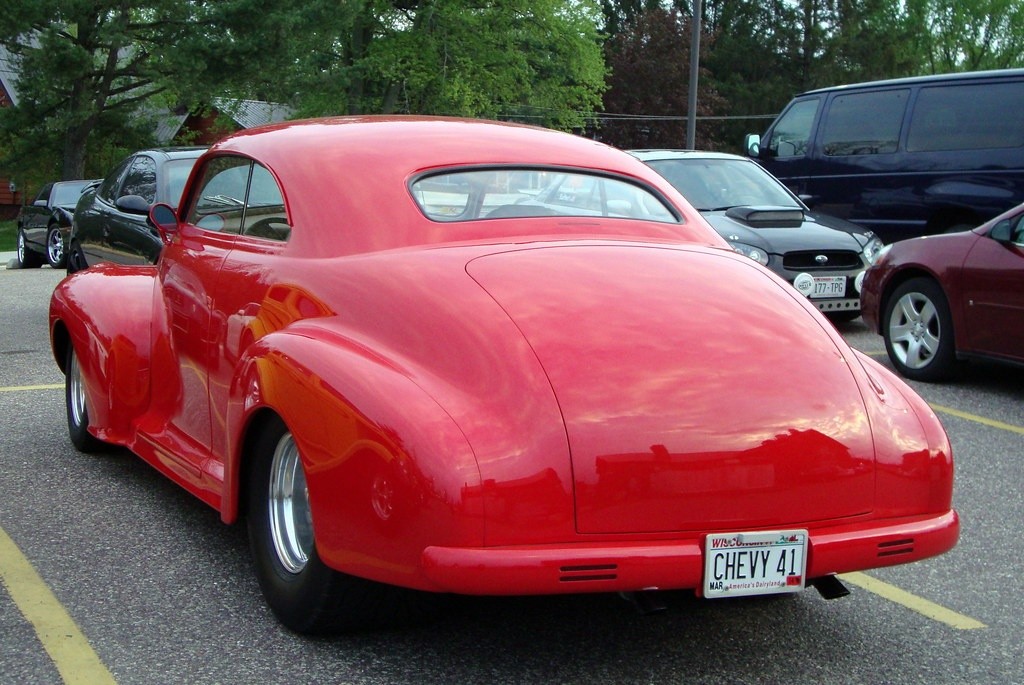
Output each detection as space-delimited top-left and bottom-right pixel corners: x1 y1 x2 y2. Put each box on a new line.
741 68 1024 247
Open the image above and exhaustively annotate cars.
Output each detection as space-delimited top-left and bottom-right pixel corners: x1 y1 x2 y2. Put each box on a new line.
860 203 1024 384
64 143 288 277
508 147 887 323
45 111 962 637
15 179 95 271
